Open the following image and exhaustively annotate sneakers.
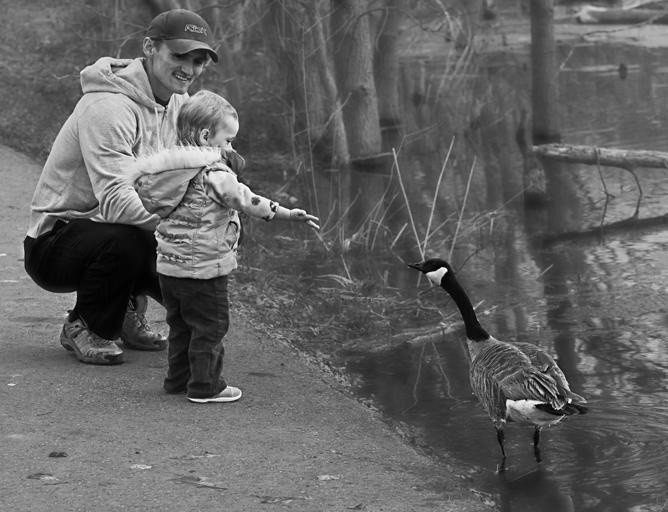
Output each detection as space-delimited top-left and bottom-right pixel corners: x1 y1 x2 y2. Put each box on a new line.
117 307 168 352
188 383 243 405
57 311 123 369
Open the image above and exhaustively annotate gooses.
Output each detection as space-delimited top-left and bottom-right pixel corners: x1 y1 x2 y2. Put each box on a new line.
407 259 588 458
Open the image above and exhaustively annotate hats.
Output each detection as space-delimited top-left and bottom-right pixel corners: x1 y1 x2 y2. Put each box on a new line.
144 8 221 65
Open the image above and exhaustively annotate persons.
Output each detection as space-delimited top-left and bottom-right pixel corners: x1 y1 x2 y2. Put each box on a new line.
131 89 321 404
24 9 220 366
